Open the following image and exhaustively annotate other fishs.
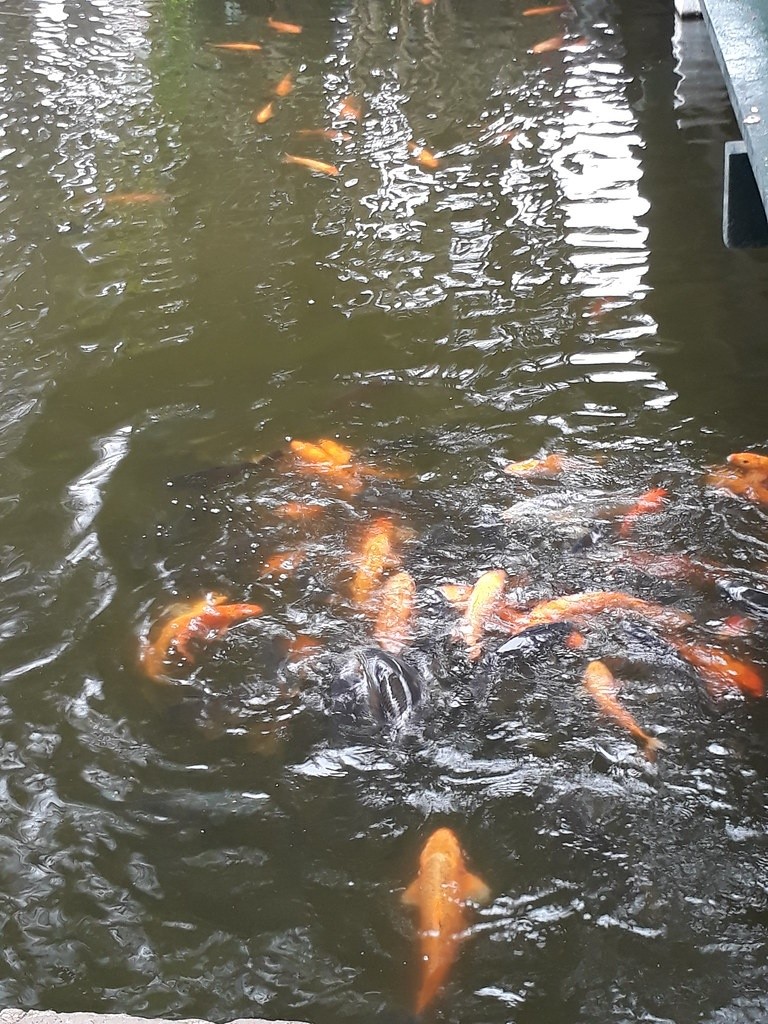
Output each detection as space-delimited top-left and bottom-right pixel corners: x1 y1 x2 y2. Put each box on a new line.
91 0 590 205
398 827 490 1018
140 436 768 763
